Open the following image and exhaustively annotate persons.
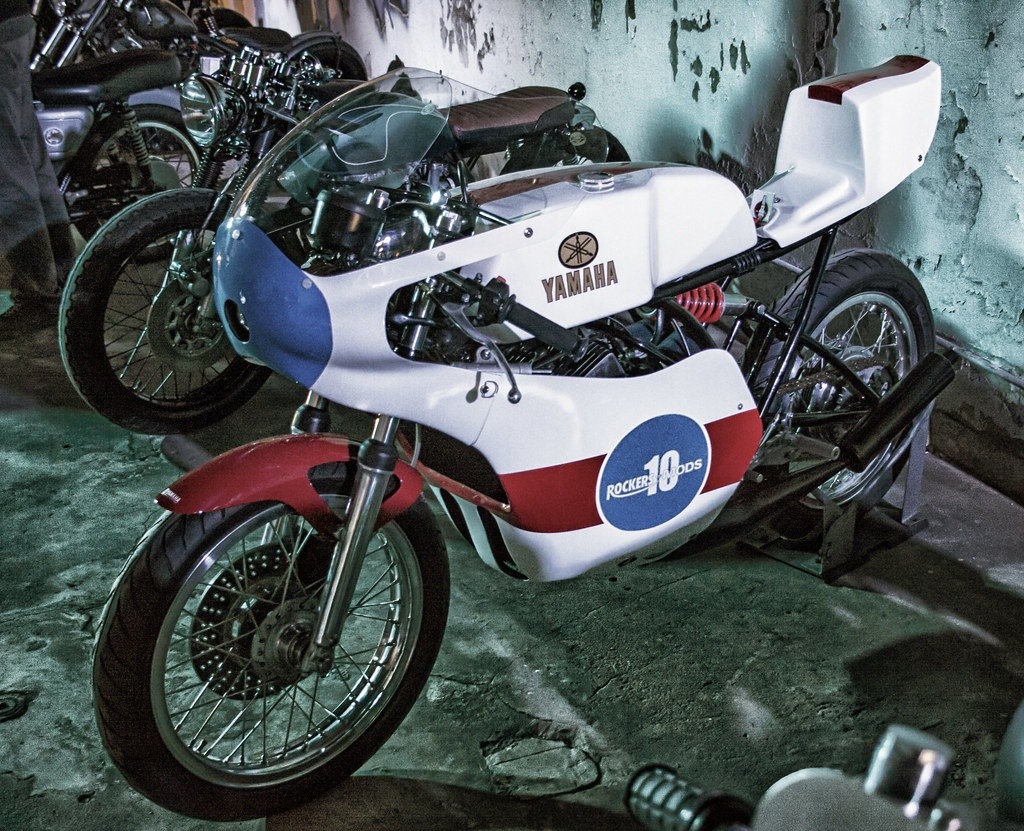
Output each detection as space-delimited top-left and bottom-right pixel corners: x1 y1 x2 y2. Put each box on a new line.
0 0 79 342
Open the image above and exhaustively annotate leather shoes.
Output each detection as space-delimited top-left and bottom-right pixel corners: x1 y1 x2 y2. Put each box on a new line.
0 295 59 340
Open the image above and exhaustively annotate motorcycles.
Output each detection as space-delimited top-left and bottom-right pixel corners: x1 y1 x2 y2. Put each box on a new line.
92 54 963 822
30 0 633 434
